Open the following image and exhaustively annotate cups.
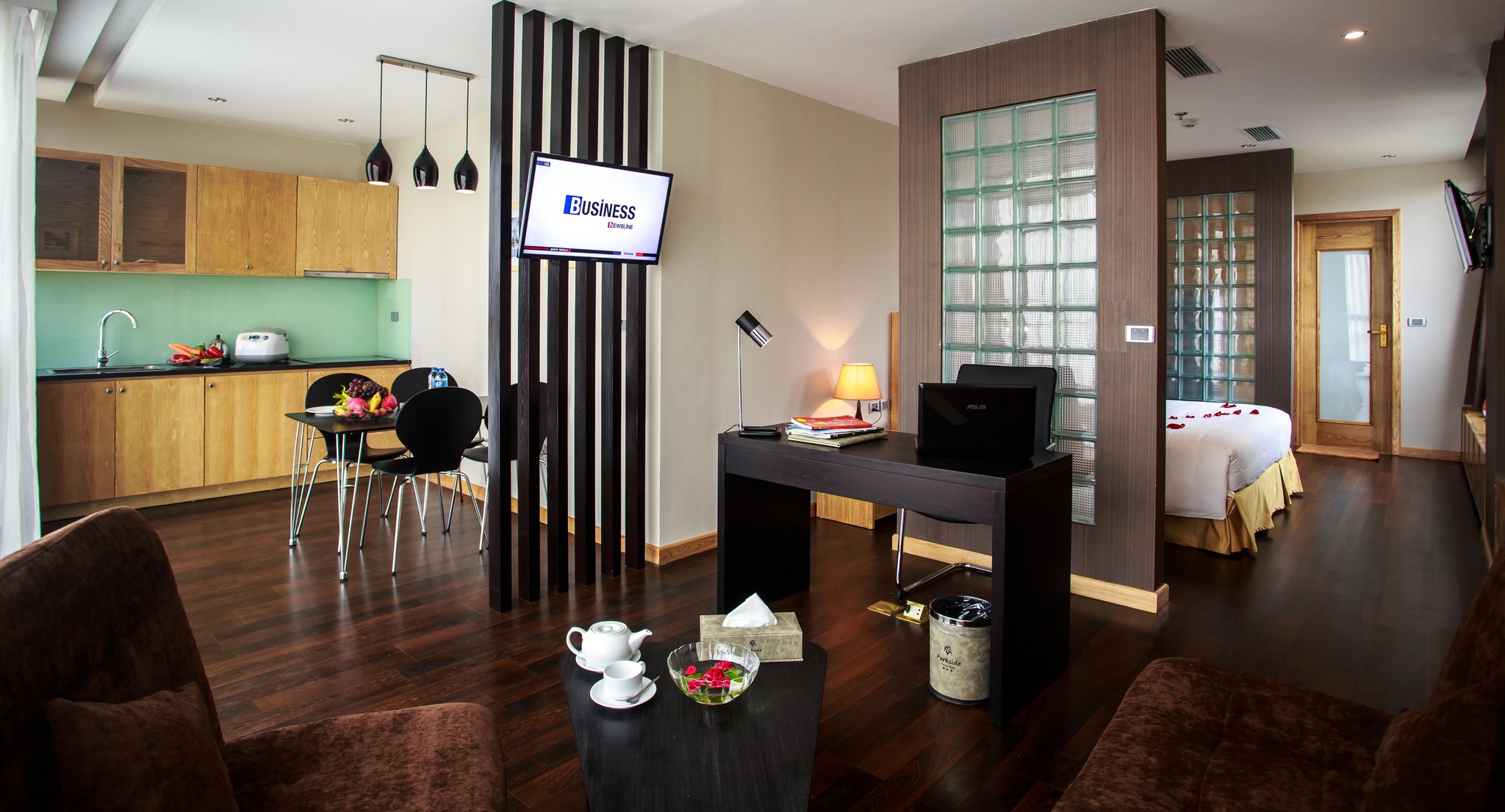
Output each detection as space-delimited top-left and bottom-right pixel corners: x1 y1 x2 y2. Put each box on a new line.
603 660 646 701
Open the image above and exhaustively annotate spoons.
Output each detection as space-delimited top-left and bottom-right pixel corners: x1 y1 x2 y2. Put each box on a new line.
627 676 660 704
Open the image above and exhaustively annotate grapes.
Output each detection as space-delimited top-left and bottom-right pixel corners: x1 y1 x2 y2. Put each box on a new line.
347 378 388 400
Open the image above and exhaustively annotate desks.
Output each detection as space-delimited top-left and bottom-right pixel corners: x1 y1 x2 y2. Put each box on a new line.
717 422 1073 729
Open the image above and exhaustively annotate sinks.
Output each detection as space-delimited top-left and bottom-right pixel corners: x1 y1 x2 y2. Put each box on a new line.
46 364 166 373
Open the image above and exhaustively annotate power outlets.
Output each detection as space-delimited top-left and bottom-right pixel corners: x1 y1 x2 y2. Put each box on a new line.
896 600 930 625
867 600 904 616
391 312 398 321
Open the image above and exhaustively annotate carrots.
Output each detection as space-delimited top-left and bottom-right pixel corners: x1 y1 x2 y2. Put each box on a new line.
168 343 204 358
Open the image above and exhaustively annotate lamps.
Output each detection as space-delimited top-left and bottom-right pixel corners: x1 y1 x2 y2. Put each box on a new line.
453 78 479 194
413 68 439 189
833 363 883 421
734 310 783 439
365 59 393 186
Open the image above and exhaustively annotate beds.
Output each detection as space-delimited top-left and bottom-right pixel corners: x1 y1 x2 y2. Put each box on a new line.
1065 394 1305 556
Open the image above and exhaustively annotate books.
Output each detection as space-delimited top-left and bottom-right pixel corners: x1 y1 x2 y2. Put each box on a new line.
783 414 889 448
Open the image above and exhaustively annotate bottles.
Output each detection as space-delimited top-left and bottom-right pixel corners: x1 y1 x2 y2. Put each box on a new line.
428 367 438 390
434 368 448 388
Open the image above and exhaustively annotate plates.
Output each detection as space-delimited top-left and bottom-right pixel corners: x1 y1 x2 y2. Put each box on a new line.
305 406 341 416
332 406 398 421
575 649 641 674
166 357 222 366
590 676 657 709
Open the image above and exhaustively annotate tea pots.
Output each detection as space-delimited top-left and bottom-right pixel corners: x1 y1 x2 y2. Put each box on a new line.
566 621 652 670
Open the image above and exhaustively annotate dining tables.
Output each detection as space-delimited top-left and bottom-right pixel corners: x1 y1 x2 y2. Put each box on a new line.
563 626 827 812
283 396 491 585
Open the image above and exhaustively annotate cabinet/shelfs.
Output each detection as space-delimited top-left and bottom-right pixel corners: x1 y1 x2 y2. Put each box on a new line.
205 371 309 499
307 430 411 483
34 147 197 274
197 165 297 278
298 176 399 281
35 376 205 523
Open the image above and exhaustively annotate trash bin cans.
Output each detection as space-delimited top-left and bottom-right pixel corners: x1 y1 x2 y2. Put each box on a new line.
926 594 993 707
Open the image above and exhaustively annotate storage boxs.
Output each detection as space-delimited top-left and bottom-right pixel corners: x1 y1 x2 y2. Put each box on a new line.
696 610 805 664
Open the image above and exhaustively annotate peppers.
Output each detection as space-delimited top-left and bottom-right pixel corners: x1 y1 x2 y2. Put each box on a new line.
171 343 224 364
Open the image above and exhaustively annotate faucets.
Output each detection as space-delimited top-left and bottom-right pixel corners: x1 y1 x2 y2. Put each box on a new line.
96 308 138 367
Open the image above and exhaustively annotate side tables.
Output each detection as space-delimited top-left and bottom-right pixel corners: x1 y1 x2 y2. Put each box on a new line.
815 491 898 529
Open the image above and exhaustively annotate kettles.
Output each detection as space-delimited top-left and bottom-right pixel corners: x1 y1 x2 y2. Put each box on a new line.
207 334 231 365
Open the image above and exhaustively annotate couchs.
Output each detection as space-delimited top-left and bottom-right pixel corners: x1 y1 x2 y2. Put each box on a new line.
0 506 508 812
1051 544 1504 812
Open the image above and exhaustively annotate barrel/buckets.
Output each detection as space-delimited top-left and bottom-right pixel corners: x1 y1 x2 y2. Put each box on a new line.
929 595 991 705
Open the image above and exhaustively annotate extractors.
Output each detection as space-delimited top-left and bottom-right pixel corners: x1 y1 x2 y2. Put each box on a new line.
304 272 390 280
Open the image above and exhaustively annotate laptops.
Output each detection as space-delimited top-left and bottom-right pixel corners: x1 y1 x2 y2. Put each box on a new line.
915 382 1037 459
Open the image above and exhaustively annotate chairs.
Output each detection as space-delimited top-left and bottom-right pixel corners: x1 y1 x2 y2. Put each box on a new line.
446 382 563 557
359 387 491 574
293 373 428 538
388 368 467 533
897 362 1057 594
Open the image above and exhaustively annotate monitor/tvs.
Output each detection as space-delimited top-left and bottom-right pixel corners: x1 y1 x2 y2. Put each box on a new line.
1443 179 1484 274
516 151 674 264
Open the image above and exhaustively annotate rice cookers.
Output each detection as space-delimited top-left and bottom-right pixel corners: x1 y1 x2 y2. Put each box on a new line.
235 328 290 364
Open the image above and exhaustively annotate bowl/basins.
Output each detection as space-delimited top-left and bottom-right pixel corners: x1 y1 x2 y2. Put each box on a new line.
667 640 760 706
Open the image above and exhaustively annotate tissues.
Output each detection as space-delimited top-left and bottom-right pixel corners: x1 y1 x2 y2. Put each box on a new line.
697 592 804 661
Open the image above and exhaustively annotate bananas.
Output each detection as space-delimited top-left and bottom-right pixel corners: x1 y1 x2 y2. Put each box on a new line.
334 407 346 416
369 392 381 413
